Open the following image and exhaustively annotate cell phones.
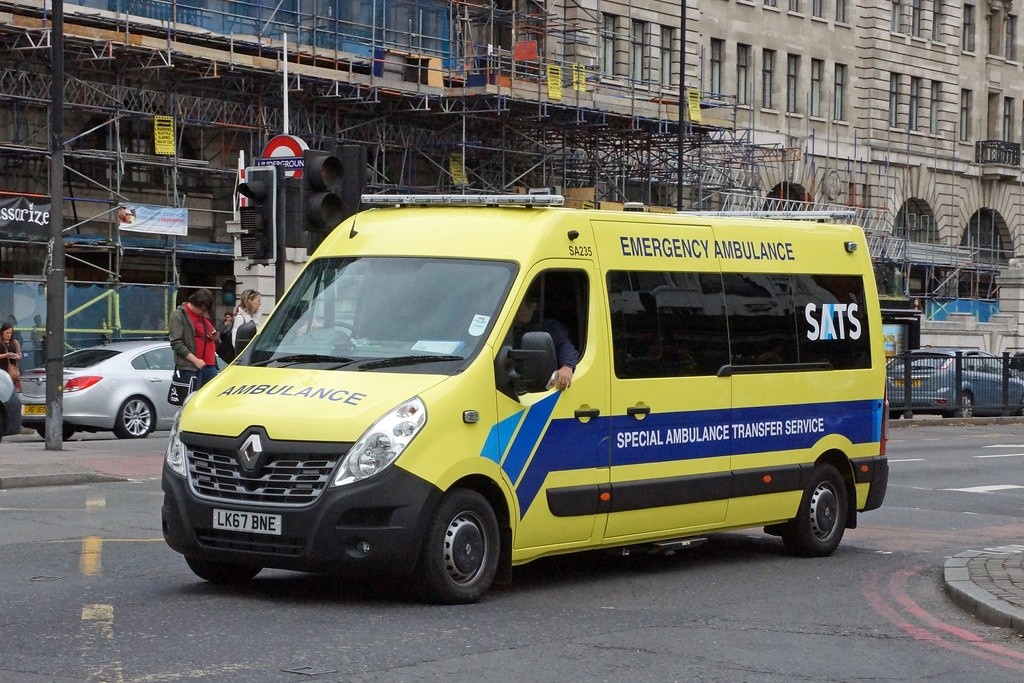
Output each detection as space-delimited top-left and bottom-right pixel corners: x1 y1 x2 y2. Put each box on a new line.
207 331 217 339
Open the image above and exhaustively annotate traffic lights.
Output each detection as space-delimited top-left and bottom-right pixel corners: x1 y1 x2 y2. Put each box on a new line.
239 168 284 267
303 147 346 256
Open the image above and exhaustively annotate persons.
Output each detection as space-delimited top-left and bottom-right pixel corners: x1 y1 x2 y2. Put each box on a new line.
7 314 46 368
0 323 23 391
218 312 232 354
514 292 579 392
166 288 220 406
232 289 261 360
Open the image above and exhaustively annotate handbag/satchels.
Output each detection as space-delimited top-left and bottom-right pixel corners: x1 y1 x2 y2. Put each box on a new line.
7 363 20 381
215 314 246 365
167 370 198 407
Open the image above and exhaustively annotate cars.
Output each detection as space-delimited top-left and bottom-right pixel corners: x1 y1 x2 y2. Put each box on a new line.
17 336 231 439
885 346 1024 420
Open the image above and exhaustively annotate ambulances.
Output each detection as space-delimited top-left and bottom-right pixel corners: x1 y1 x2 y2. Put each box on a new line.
158 191 892 606
